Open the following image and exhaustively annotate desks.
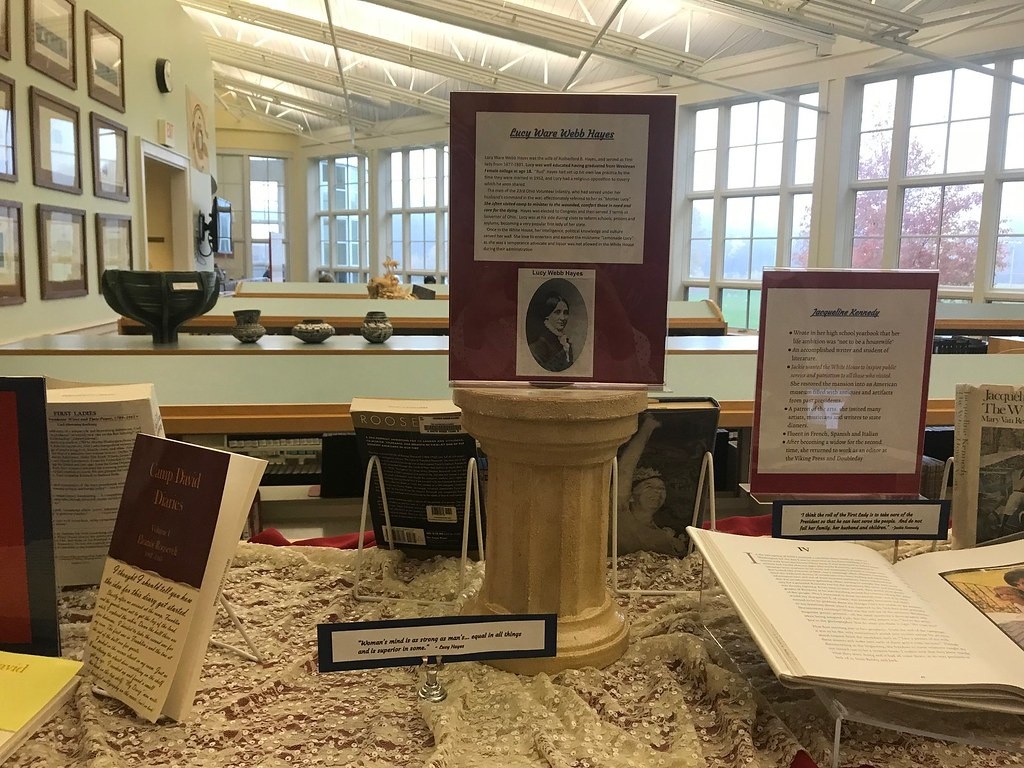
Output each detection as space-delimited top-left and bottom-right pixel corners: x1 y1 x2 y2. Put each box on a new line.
1 283 1024 455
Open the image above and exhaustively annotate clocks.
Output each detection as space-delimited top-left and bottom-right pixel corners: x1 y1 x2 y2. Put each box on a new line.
156 59 173 92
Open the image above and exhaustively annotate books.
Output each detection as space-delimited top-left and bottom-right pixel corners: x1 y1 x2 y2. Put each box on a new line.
0 376 1023 767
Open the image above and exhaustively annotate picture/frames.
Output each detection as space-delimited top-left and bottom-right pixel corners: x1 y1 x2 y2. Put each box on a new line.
0 0 135 304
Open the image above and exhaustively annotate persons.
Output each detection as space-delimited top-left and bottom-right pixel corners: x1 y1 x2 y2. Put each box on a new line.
530 296 573 372
609 416 686 556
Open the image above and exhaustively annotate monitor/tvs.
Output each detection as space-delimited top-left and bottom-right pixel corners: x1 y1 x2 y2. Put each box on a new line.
210 196 233 254
